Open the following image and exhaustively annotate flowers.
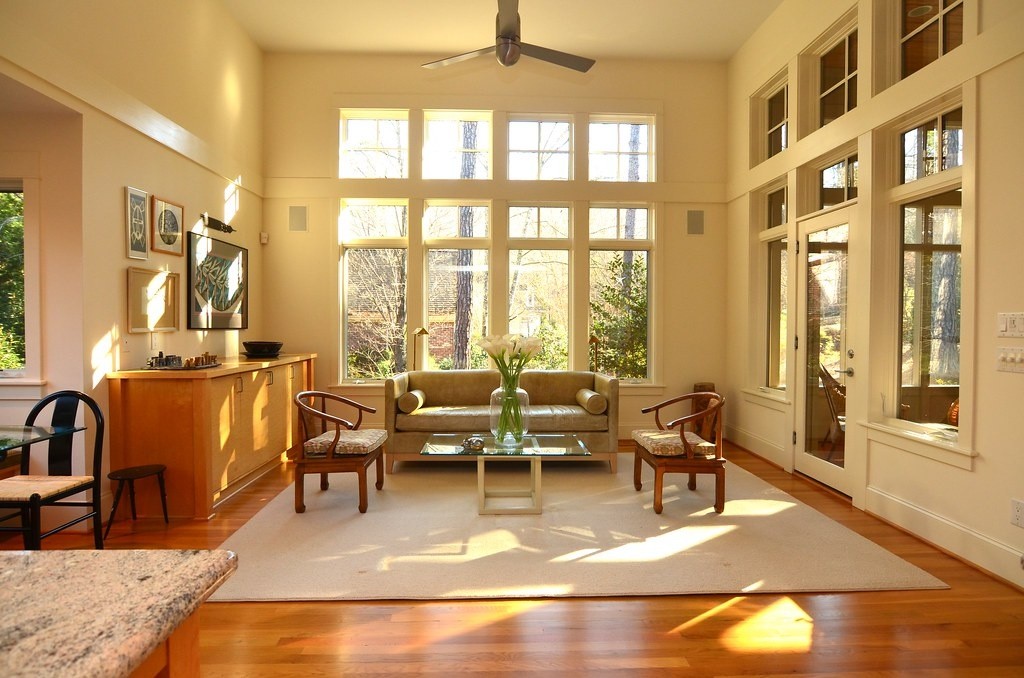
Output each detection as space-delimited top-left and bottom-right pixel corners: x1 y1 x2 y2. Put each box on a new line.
474 333 543 440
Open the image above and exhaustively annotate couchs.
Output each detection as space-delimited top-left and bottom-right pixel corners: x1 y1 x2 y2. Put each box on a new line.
383 368 619 474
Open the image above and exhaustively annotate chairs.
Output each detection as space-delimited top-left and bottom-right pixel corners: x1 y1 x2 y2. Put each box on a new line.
294 390 388 514
811 364 910 462
0 390 104 550
631 391 727 515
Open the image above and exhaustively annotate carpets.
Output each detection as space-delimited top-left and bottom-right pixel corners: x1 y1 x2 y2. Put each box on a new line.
202 459 952 603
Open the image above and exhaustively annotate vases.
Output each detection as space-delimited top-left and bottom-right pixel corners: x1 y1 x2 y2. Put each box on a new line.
487 369 529 447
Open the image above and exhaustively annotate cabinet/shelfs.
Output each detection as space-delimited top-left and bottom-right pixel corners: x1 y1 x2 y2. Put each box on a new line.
105 352 318 519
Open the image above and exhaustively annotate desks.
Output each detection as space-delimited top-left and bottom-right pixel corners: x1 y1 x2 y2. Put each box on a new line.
0 424 87 463
0 548 239 678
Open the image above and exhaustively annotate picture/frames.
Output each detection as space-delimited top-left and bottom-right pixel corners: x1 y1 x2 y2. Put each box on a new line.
124 186 248 334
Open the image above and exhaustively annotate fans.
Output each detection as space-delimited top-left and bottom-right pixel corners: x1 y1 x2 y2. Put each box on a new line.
422 0 596 73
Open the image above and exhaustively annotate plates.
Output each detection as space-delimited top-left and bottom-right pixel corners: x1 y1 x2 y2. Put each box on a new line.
241 352 280 358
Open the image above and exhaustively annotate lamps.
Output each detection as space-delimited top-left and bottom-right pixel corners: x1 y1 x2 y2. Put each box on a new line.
589 336 600 371
411 328 429 371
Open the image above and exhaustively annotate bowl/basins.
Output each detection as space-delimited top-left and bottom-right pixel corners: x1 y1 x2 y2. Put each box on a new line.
243 341 283 353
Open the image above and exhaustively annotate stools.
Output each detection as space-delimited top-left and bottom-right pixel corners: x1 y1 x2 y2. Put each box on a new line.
104 464 168 541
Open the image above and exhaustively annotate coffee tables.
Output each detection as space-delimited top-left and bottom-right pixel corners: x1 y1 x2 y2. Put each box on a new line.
419 433 593 516
